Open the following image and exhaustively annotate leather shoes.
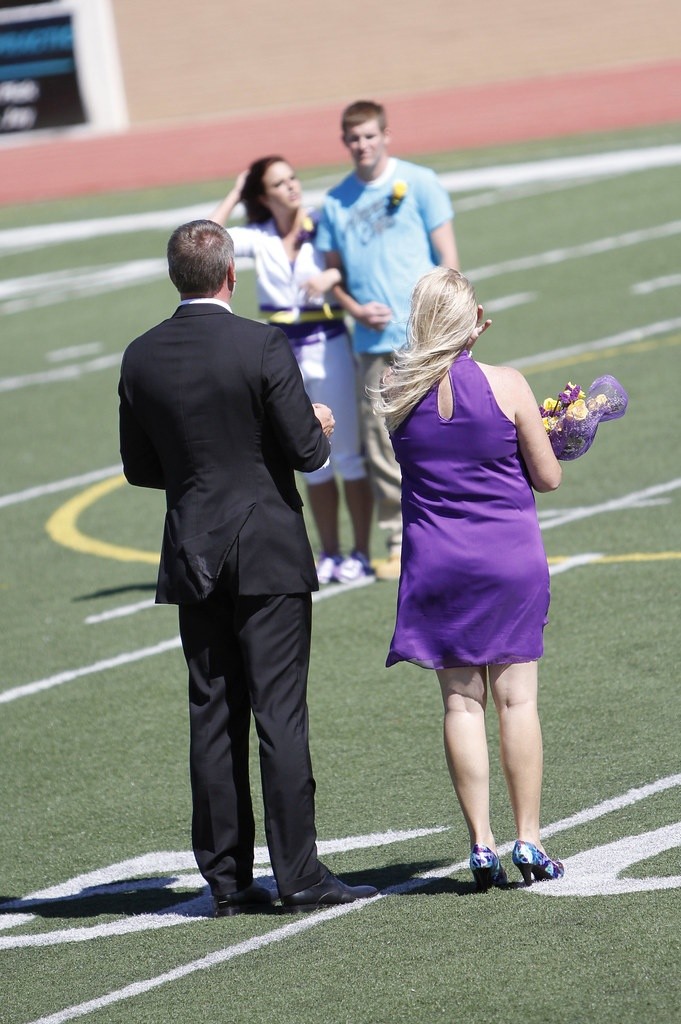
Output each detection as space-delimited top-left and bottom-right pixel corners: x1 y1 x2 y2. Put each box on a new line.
283 870 377 915
216 881 282 916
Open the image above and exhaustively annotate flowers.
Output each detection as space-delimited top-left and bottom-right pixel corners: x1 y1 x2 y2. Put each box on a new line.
539 374 629 461
391 180 409 205
292 211 321 241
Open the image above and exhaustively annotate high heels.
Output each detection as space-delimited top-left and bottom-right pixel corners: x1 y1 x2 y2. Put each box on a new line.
511 839 564 887
470 843 508 893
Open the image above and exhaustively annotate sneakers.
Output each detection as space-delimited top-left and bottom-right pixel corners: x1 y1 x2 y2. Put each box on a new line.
333 552 375 584
316 554 342 585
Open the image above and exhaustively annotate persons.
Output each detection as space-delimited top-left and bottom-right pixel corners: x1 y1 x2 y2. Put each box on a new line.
117 218 380 916
315 100 460 580
364 265 565 890
207 156 378 584
